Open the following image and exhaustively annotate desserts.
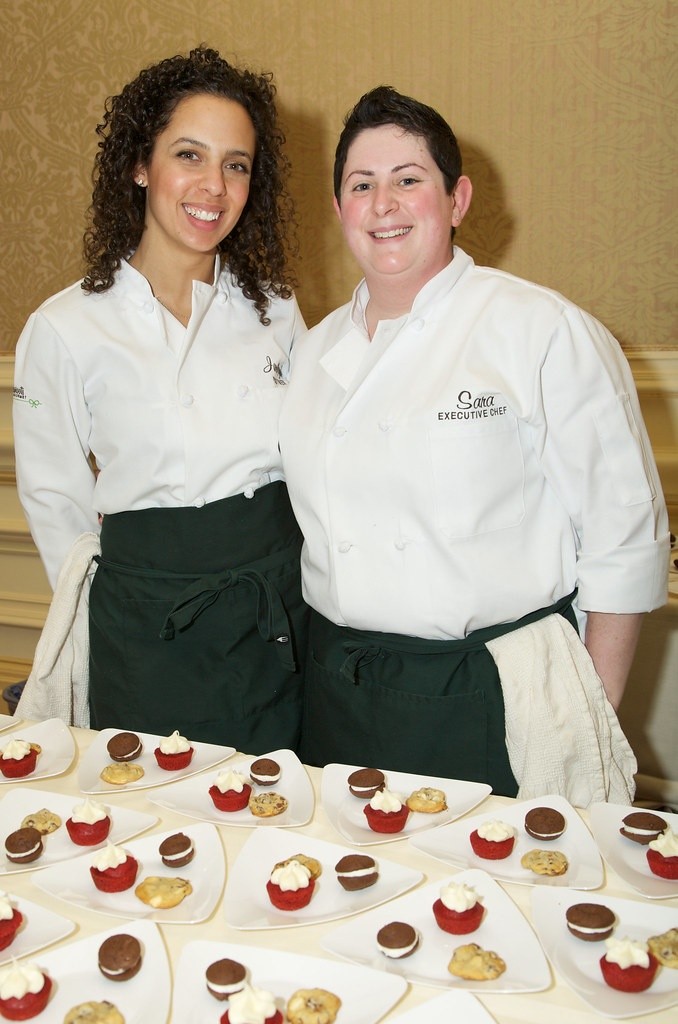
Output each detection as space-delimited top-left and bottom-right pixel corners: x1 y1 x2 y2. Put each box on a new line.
0 730 678 1024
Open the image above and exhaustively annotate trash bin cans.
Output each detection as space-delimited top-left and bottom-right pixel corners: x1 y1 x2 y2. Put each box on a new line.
2 678 28 716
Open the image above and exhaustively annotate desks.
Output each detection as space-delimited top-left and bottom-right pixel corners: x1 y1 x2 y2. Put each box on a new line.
0 715 675 1021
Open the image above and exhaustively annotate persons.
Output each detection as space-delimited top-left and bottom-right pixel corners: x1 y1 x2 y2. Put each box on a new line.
278 82 671 804
15 42 308 757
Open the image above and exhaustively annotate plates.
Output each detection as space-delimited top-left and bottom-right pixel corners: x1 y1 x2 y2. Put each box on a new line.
0 713 677 1023
